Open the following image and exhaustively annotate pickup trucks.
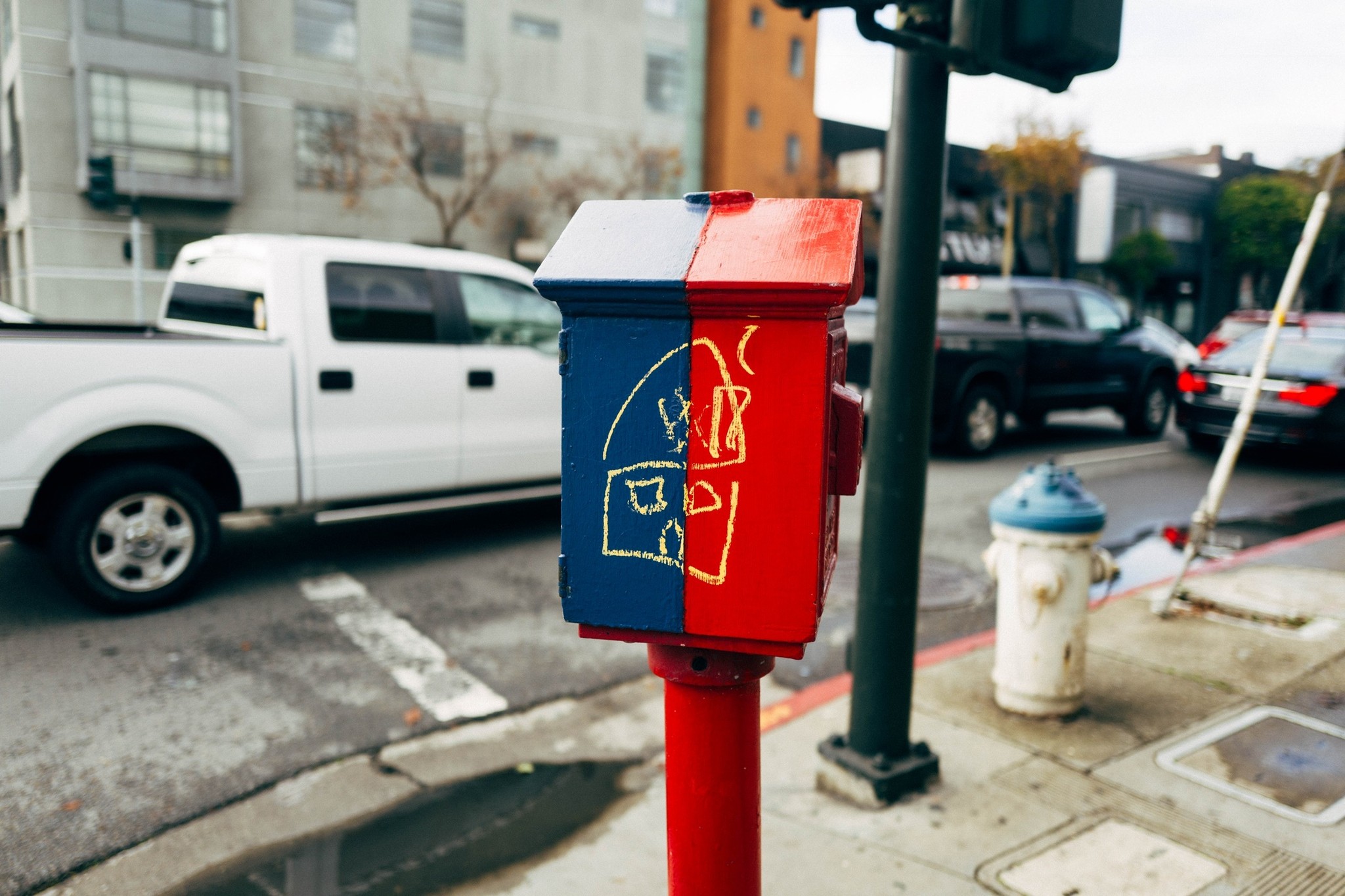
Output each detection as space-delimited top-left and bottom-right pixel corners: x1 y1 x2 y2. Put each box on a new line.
845 283 1177 464
1 230 567 618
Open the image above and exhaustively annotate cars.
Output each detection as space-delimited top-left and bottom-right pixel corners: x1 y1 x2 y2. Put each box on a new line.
844 273 1345 470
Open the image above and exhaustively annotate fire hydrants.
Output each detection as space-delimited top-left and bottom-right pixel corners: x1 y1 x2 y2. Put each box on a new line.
979 455 1121 717
